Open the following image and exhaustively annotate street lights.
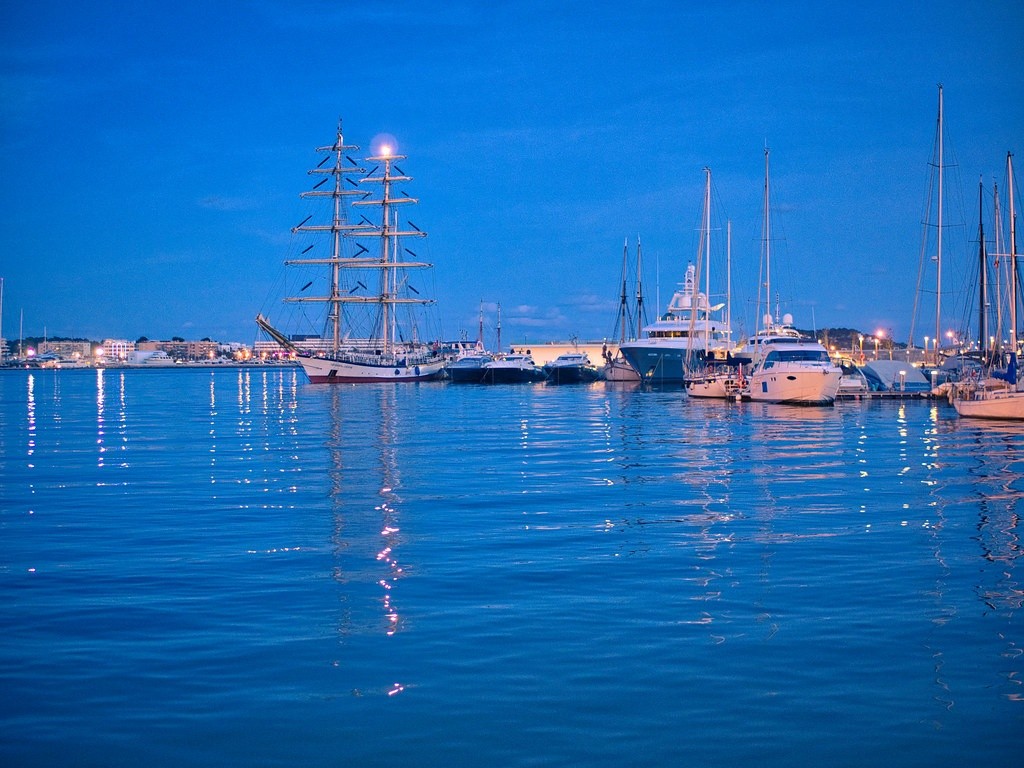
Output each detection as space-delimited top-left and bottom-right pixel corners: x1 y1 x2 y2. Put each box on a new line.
990 335 994 349
932 339 937 365
859 335 865 355
924 336 930 364
873 338 880 360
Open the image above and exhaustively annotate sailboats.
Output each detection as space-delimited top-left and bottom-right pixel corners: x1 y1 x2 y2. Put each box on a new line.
254 117 458 384
902 84 991 386
681 166 748 398
750 137 843 405
950 149 1024 420
0 277 68 370
601 234 650 381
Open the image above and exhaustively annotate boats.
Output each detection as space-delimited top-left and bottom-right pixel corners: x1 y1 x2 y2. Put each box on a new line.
482 351 542 383
145 350 174 364
444 349 495 382
617 261 737 392
541 350 599 380
